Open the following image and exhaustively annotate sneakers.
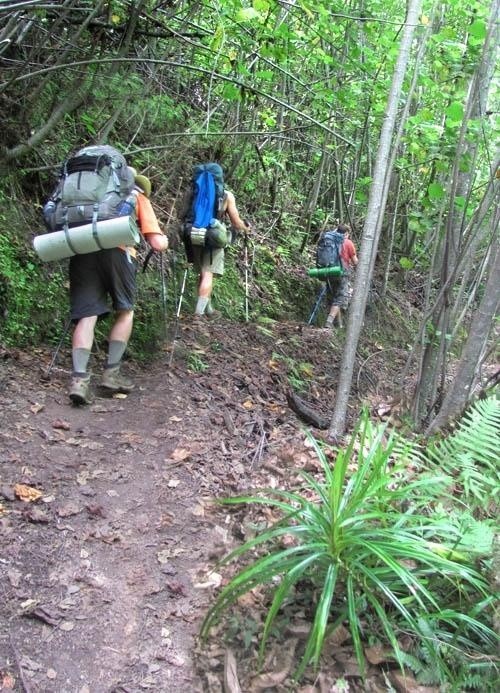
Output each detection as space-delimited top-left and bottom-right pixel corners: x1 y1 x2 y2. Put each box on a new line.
192 313 215 326
207 309 222 320
99 364 136 391
68 374 96 405
324 321 336 329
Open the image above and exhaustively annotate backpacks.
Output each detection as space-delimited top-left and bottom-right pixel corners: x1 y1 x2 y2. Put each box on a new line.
315 230 344 269
41 143 141 231
178 163 227 229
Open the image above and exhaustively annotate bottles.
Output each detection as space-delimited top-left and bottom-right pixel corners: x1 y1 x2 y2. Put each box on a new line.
111 188 140 218
209 218 227 232
43 200 56 223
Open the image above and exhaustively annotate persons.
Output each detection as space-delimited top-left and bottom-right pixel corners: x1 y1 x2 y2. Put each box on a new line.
174 181 245 318
68 174 168 402
315 222 359 329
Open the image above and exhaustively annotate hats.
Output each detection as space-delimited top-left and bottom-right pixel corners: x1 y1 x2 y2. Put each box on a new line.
126 164 152 198
